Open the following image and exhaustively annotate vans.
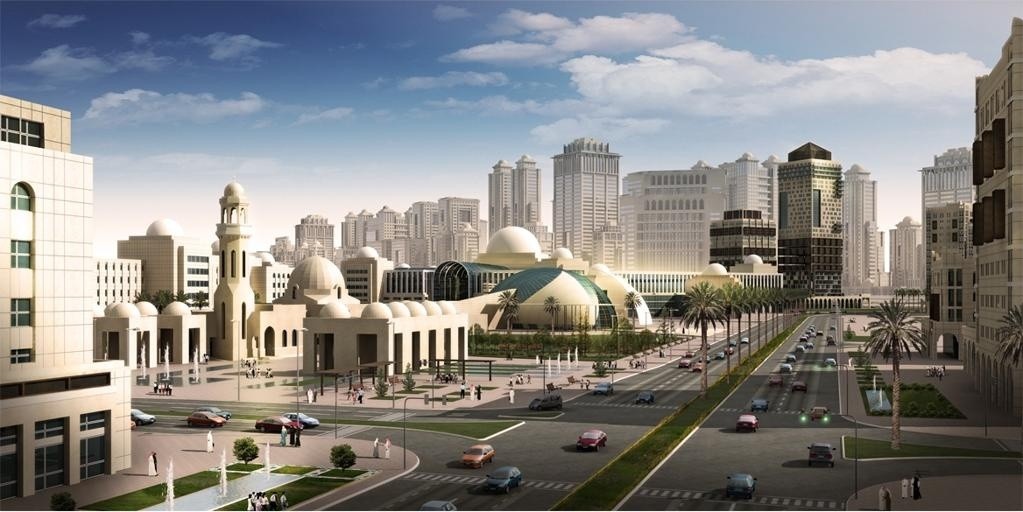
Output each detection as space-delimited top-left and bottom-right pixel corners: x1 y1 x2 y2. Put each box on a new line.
530 392 563 411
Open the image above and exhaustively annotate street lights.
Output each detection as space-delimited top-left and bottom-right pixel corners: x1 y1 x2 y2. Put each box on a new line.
668 308 680 361
230 318 241 401
295 328 309 429
643 311 655 369
609 314 625 387
385 321 398 408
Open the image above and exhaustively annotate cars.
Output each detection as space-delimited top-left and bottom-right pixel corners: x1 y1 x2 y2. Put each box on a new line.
591 334 749 404
768 376 784 386
810 406 830 421
750 399 769 413
791 381 808 393
577 428 606 451
780 321 836 373
736 413 759 433
724 473 757 498
130 405 321 436
483 466 523 494
420 501 458 511
461 444 495 469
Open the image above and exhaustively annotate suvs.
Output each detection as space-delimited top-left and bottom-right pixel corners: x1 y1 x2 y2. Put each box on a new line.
806 443 837 468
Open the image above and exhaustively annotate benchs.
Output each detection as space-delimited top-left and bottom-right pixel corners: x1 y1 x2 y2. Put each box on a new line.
388 376 403 386
440 375 457 384
567 376 580 385
546 382 562 394
350 384 364 392
